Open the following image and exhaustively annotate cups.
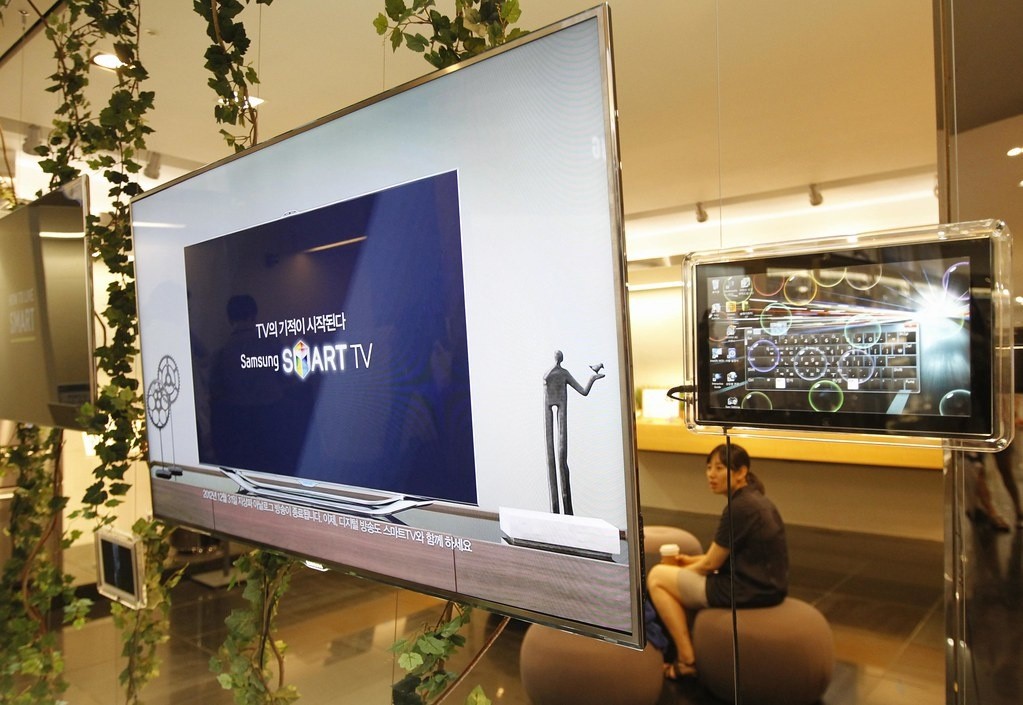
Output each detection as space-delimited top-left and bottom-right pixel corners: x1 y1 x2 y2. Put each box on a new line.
659 544 680 566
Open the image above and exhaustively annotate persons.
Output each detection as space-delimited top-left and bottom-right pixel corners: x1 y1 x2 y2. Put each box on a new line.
646 443 790 685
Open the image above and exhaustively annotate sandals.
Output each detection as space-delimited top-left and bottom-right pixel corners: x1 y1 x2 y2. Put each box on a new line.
664 660 698 681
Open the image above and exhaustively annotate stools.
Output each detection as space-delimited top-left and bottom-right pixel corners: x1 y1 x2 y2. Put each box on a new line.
641 524 705 589
519 621 665 705
691 596 836 705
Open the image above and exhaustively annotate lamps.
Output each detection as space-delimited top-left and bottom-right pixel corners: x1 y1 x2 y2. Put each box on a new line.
808 183 823 206
694 202 708 223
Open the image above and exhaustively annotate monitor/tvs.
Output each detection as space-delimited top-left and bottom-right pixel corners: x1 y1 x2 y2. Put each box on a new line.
93 529 145 611
128 2 640 650
0 173 97 435
681 220 1013 453
